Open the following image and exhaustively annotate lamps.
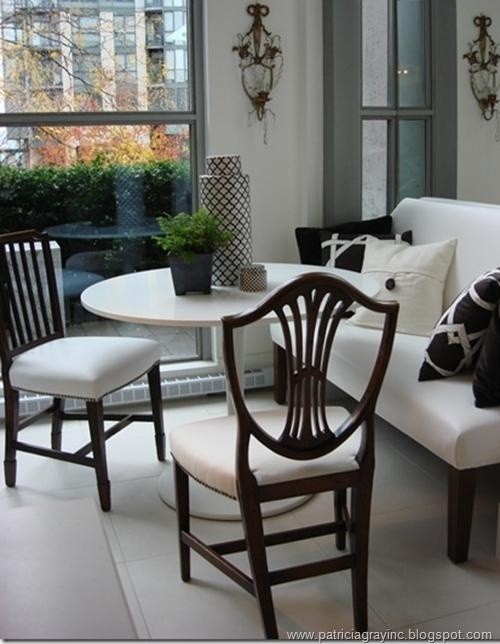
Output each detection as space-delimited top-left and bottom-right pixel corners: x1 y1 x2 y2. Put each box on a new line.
229 1 287 143
462 11 500 142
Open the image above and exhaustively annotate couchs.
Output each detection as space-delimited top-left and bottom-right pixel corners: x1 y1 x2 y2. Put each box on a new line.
268 188 498 565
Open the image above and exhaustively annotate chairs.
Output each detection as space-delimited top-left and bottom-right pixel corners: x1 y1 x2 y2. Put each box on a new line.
168 270 401 641
0 228 166 513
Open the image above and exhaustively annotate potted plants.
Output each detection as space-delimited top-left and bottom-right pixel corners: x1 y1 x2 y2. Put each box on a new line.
150 210 242 295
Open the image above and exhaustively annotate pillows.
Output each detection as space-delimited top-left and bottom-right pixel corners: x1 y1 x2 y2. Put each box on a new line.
287 213 500 409
